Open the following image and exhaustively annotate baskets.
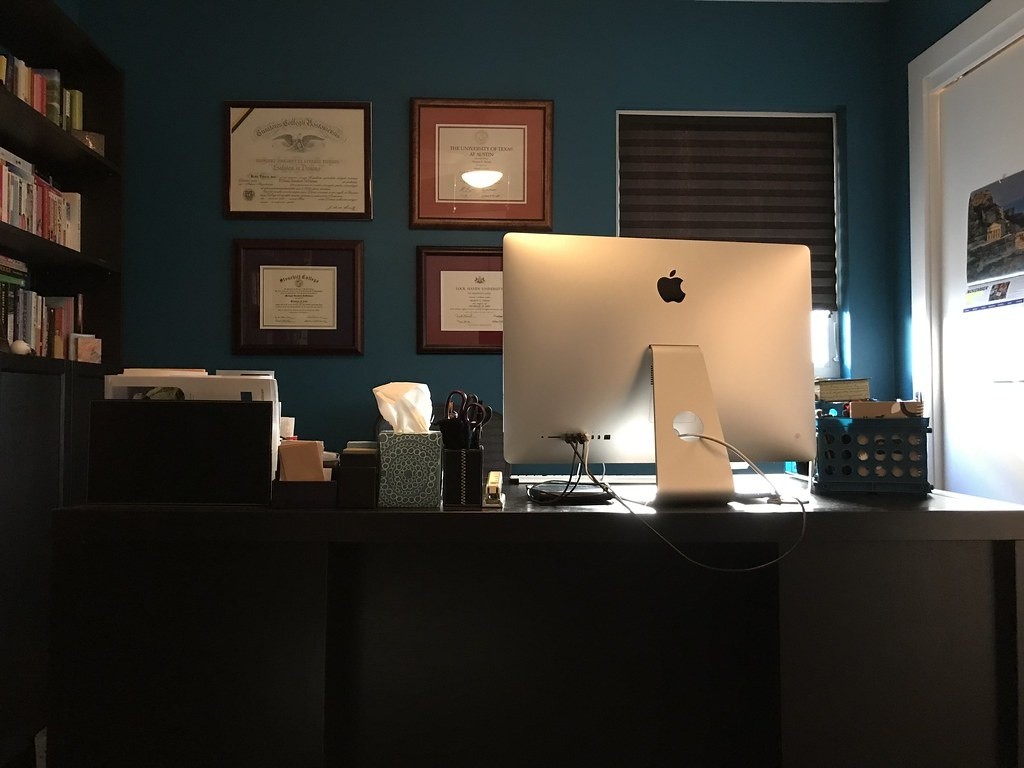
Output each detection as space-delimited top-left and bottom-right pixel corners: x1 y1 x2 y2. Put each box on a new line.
814 399 933 499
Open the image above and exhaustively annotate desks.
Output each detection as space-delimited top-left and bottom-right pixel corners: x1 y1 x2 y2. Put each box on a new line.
50 475 1024 768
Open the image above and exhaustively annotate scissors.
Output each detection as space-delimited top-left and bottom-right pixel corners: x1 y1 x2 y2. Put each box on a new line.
444 389 493 449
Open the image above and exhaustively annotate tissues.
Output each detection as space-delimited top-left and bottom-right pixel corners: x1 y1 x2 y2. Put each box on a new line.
372 382 442 510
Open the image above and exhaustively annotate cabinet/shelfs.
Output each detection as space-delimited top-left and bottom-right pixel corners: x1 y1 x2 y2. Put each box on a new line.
0 0 126 768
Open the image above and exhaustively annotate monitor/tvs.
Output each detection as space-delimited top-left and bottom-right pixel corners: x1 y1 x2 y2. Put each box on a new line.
503 233 817 510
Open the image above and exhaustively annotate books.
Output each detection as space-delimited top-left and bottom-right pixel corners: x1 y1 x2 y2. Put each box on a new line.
852 398 924 477
0 52 83 138
0 256 101 364
816 376 870 401
0 146 82 252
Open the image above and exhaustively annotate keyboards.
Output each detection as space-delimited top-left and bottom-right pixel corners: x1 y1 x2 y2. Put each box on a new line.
510 474 657 484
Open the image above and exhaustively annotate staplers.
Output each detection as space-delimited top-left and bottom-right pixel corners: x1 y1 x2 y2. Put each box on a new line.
482 470 506 510
